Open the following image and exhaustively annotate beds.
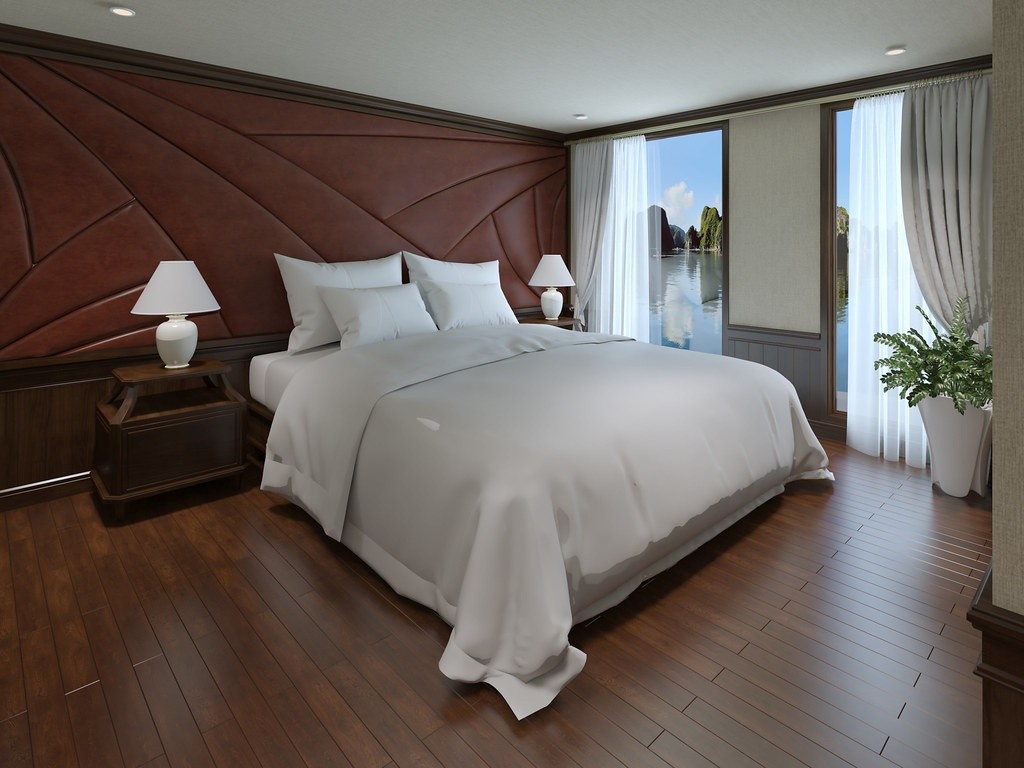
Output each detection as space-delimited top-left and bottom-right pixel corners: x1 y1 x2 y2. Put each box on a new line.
248 324 835 722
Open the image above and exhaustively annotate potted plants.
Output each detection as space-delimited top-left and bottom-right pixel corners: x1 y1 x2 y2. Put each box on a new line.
873 296 996 500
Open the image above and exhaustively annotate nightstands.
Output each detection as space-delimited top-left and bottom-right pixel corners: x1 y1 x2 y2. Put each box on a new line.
517 317 581 330
90 358 247 520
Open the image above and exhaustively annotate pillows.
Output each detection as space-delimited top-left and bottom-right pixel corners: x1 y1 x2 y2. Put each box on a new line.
273 251 402 356
420 279 520 331
403 250 502 324
317 282 438 351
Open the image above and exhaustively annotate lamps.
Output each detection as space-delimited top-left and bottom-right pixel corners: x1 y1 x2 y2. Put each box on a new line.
129 260 221 370
528 255 576 321
